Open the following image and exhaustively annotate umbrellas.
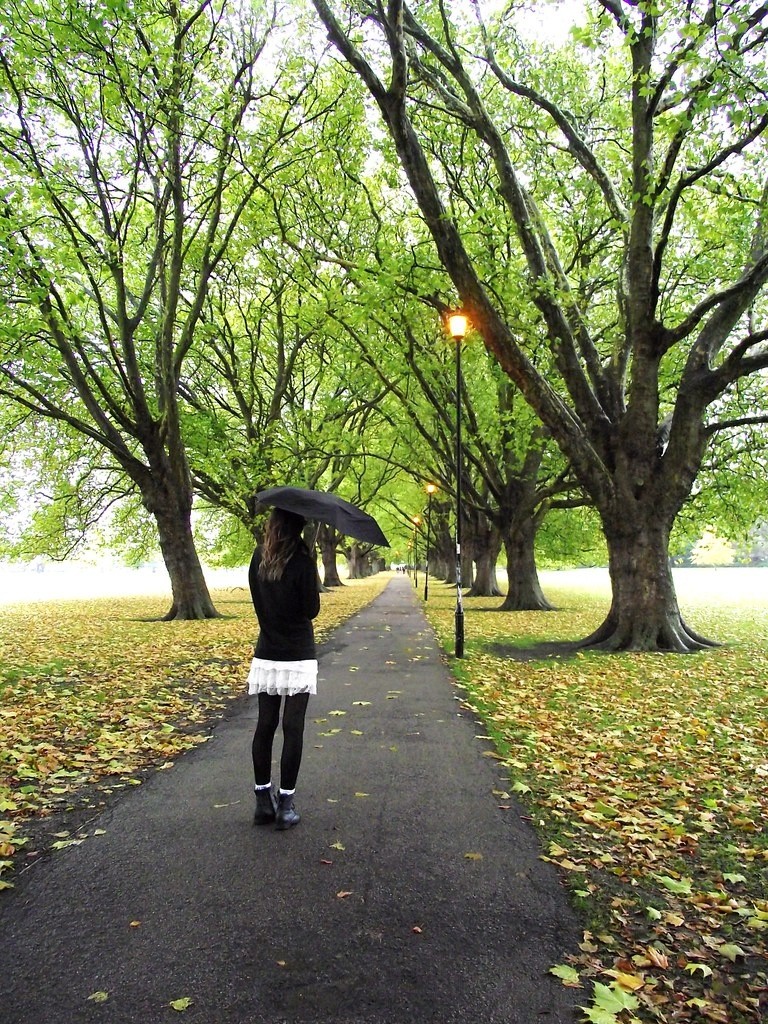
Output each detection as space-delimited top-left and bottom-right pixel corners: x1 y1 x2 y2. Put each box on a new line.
250 486 392 548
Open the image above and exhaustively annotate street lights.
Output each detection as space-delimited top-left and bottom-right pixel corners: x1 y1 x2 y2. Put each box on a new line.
415 516 419 587
407 529 416 580
424 482 436 602
447 307 469 659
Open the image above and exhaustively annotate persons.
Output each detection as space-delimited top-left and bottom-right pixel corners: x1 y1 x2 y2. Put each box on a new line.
246 507 321 831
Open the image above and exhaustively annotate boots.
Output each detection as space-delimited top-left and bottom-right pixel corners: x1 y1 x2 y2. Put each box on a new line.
253 785 276 826
275 789 301 830
